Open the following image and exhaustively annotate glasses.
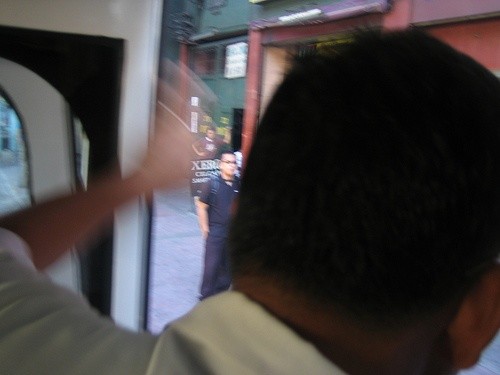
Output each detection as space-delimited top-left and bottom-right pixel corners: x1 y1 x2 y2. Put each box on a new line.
220 160 237 164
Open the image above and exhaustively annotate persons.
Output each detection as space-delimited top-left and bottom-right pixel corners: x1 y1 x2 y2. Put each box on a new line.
192 126 232 158
0 24 500 375
194 151 242 301
